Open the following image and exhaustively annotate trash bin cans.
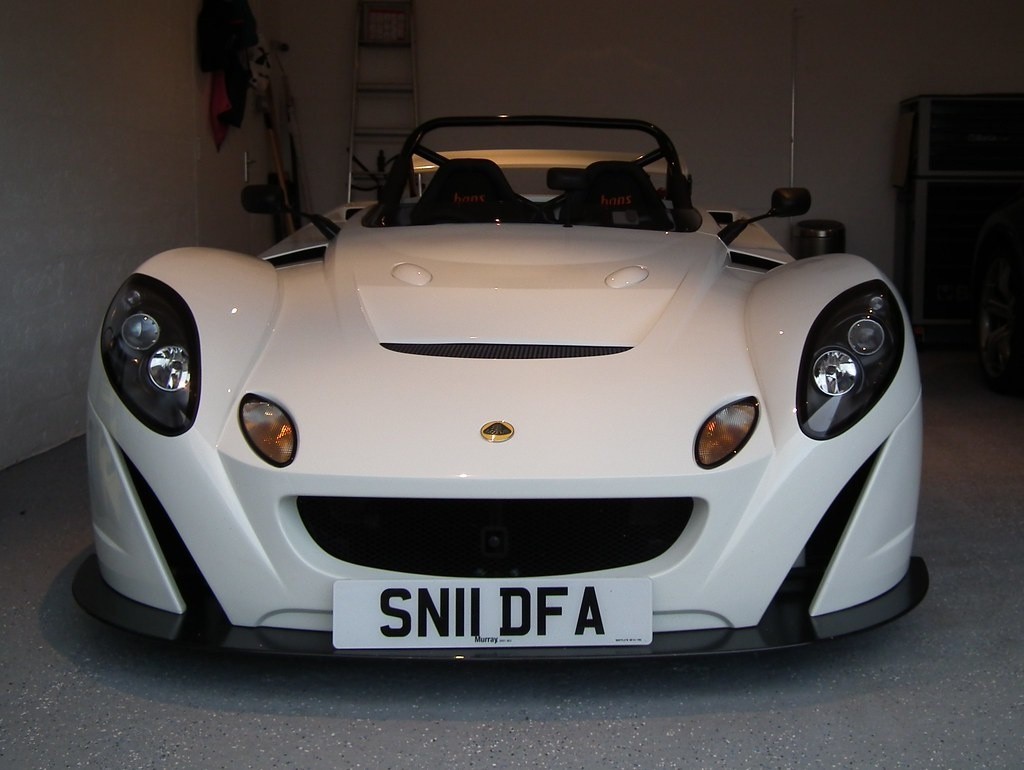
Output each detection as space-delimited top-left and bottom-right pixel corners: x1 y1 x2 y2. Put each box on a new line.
793 220 846 259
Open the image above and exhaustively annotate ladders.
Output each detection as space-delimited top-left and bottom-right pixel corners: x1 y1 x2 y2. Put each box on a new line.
346 1 423 205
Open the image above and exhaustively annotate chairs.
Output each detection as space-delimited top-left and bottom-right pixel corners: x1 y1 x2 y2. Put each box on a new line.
562 149 677 230
412 156 551 223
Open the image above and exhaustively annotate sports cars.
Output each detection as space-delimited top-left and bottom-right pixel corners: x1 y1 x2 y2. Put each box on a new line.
81 113 935 668
